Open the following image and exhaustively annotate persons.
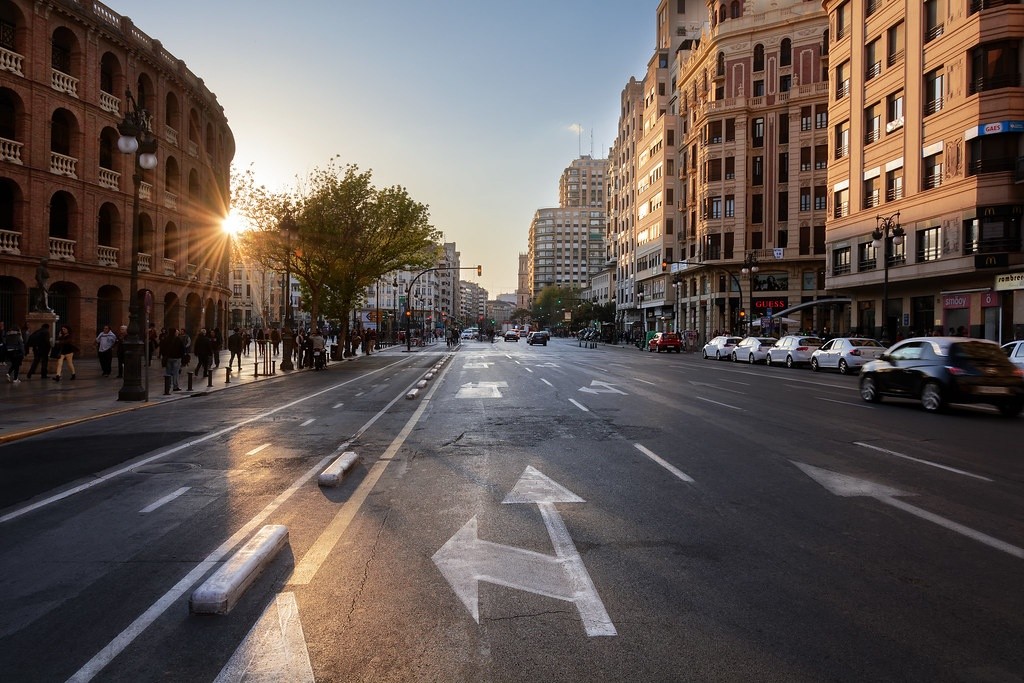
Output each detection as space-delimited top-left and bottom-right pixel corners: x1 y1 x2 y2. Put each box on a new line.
804 274 814 289
754 275 788 291
0 321 1024 391
31 258 54 313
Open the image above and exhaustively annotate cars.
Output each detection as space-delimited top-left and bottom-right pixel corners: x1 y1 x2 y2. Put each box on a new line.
461 328 481 339
810 337 888 375
702 335 779 365
647 332 682 354
858 336 1024 418
504 329 550 346
766 335 825 368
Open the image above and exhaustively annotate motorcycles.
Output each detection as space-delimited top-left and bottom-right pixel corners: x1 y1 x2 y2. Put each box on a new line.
313 346 329 372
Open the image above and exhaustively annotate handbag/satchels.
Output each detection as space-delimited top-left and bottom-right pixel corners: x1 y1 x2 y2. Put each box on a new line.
50 343 61 358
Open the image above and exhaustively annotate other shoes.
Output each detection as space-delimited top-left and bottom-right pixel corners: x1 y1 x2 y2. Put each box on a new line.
56 375 60 381
203 374 209 377
6 373 11 383
13 379 21 382
195 369 198 376
27 373 31 378
70 375 76 380
173 388 182 392
41 375 50 379
117 375 123 379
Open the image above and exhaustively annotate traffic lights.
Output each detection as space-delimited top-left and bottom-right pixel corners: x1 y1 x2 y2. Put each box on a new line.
662 259 666 271
741 308 745 320
478 265 481 276
406 310 411 317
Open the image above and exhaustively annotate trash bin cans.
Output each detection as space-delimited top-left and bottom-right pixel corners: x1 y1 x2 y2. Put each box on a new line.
331 344 338 358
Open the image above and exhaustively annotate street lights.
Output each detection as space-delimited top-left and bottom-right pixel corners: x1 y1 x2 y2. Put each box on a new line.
611 292 618 345
636 284 644 342
672 270 683 332
741 248 760 334
278 206 300 371
872 210 904 347
374 275 398 350
413 290 433 346
117 83 159 402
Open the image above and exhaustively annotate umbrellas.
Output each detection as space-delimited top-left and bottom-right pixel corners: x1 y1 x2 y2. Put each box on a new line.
743 316 798 325
